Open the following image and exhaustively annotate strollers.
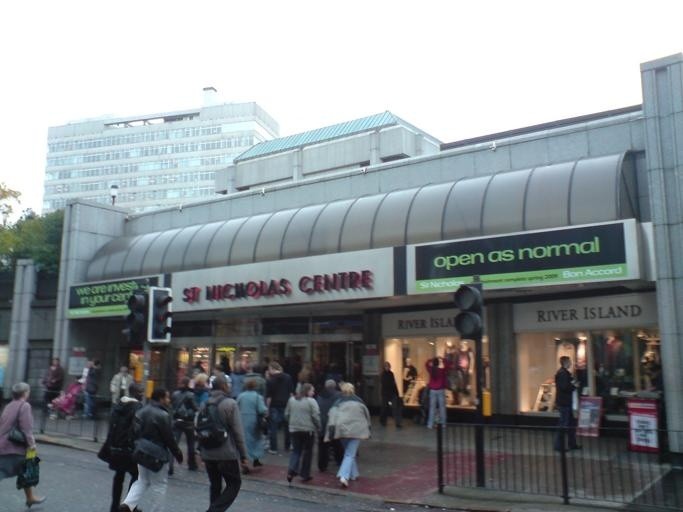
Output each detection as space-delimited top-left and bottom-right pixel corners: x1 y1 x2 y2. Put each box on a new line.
45 378 84 421
412 384 435 428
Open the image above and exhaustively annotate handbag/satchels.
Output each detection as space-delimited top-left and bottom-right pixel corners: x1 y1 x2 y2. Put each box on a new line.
109 446 132 472
16 457 40 488
259 418 270 435
177 409 195 431
9 426 26 443
132 438 168 472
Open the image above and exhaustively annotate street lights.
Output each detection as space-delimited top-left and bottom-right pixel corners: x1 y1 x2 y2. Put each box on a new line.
110 183 119 206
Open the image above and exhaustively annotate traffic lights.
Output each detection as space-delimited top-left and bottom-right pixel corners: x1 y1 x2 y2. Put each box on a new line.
453 280 483 340
148 286 174 342
121 287 149 349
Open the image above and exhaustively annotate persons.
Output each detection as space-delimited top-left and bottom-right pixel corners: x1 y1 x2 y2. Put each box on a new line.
193 376 250 512
554 356 583 453
379 361 402 432
190 356 294 474
168 377 200 475
97 383 145 512
42 357 64 405
0 382 46 508
403 340 470 393
110 365 133 404
283 363 369 488
118 389 182 512
425 356 451 429
78 359 103 418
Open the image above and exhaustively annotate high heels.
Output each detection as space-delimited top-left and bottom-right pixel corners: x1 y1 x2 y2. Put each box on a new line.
26 495 46 508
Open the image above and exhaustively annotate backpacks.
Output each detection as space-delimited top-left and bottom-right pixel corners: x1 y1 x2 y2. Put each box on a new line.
196 397 228 449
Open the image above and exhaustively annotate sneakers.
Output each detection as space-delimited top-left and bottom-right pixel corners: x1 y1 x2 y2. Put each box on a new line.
554 443 569 451
242 459 262 473
569 445 583 449
288 462 359 488
118 504 142 512
